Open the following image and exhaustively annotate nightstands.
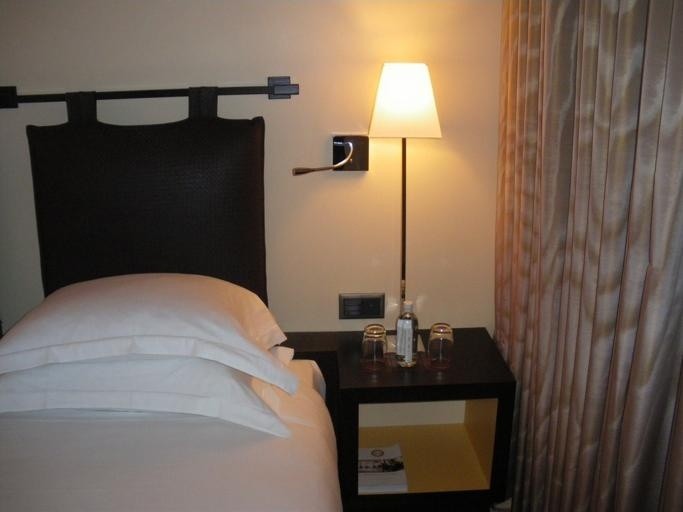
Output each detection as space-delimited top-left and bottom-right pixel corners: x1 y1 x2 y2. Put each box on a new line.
335 327 516 511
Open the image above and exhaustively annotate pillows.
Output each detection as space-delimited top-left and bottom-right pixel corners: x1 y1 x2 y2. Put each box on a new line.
1 343 297 441
1 272 301 396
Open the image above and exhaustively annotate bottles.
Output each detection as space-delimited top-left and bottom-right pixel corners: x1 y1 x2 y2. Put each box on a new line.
394 300 418 367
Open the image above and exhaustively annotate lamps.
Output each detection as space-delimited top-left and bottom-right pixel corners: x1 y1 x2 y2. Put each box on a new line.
367 62 442 354
292 135 368 175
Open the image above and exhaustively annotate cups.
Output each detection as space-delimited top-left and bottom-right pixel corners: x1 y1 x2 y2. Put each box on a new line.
426 322 455 370
357 323 389 374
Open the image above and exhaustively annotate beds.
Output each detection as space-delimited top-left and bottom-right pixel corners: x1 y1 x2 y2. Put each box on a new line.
0 77 344 512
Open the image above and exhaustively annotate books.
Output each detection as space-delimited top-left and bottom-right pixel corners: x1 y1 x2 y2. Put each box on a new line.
358 445 410 495
